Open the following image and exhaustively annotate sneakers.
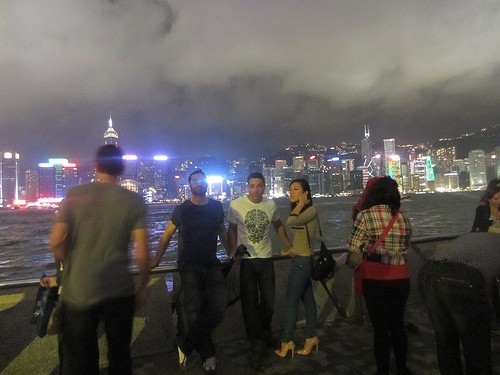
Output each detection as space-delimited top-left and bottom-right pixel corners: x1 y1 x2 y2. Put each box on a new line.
202 356 221 375
175 342 190 373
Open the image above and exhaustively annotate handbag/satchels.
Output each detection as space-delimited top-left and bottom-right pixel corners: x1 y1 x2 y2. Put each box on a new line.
309 242 336 281
354 260 363 297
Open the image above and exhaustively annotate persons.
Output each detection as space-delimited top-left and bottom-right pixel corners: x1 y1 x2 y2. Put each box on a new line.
226 173 293 369
48 144 152 375
274 178 320 358
152 169 230 371
347 176 500 375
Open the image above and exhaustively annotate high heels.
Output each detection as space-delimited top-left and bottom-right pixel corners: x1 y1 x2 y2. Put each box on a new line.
295 337 320 356
274 340 295 359
